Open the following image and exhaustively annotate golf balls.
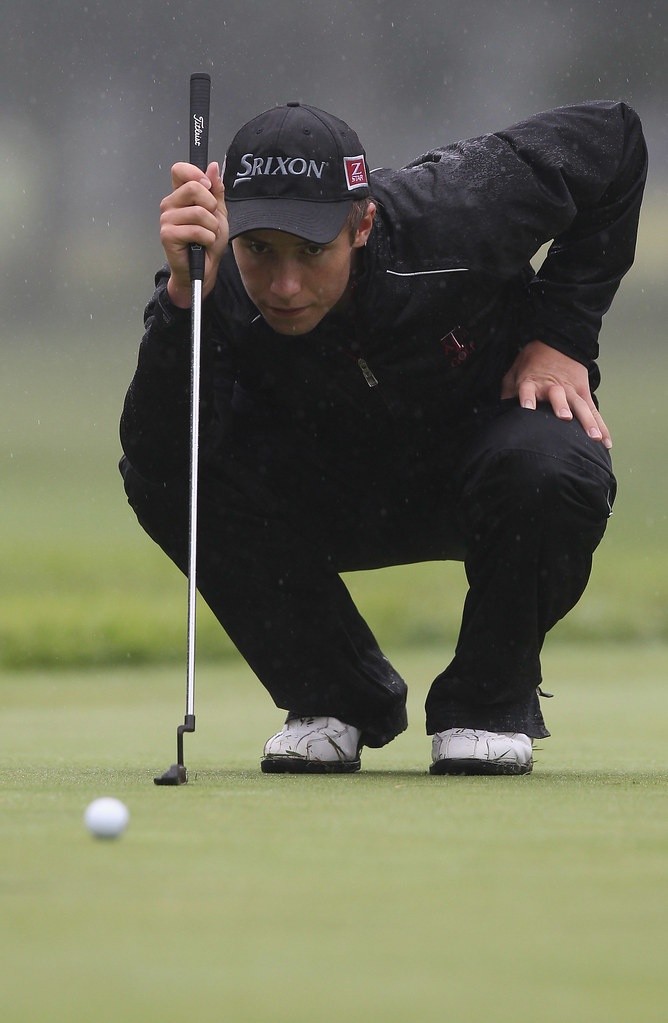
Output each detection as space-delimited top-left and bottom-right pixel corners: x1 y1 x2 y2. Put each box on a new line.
85 796 130 840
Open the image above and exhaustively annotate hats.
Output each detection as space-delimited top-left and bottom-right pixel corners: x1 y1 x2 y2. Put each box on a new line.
220 101 371 244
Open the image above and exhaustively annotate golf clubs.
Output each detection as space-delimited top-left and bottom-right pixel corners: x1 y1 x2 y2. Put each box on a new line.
153 72 212 785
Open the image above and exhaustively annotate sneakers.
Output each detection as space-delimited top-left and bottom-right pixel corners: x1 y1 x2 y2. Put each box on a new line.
260 712 363 773
427 728 534 773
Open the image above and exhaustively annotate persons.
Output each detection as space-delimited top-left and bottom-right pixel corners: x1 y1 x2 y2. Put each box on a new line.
117 101 651 776
